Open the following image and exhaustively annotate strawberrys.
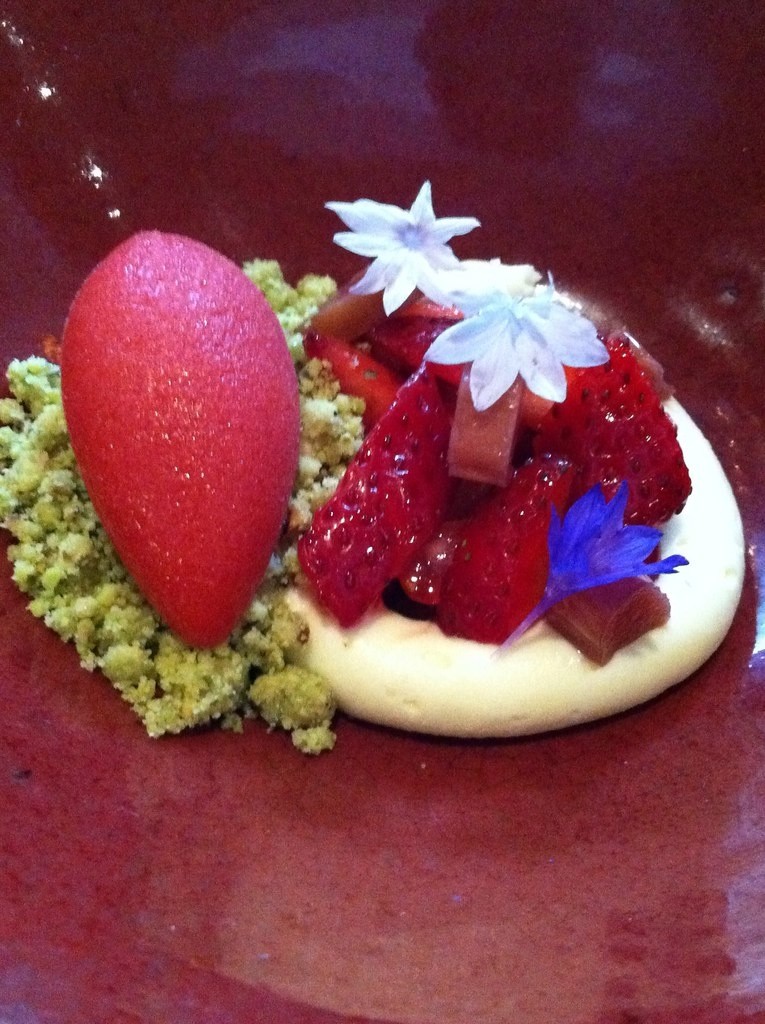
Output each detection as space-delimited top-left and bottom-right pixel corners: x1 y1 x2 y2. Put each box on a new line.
299 261 692 646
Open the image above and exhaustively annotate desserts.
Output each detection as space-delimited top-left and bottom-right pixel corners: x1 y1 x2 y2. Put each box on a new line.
1 228 368 756
276 178 745 738
